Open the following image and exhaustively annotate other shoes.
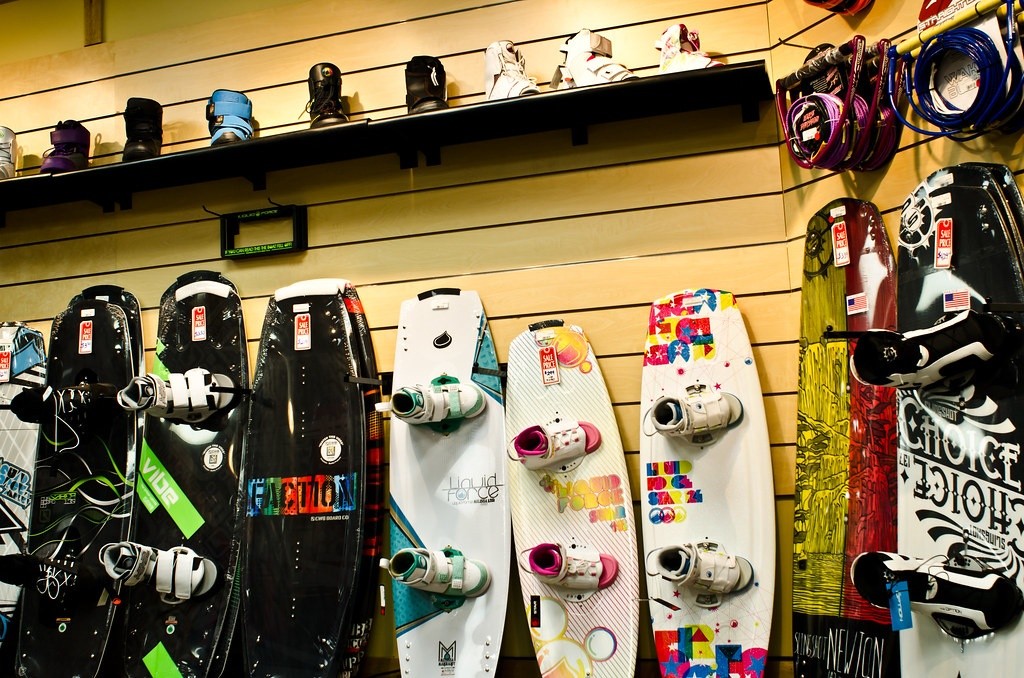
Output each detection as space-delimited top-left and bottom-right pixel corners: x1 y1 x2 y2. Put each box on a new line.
849 308 1003 388
98 541 217 598
375 383 487 424
507 419 601 471
10 383 118 423
519 543 617 590
380 548 492 598
117 367 236 423
850 550 1024 631
643 393 742 436
647 543 754 593
0 554 108 601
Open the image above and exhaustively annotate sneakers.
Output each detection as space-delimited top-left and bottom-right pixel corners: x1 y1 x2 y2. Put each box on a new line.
0 24 726 182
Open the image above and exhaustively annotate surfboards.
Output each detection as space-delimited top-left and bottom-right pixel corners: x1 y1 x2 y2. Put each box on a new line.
791 196 905 678
504 319 639 678
639 283 778 678
118 270 251 678
843 162 1024 678
240 278 385 678
13 284 147 678
388 286 511 678
